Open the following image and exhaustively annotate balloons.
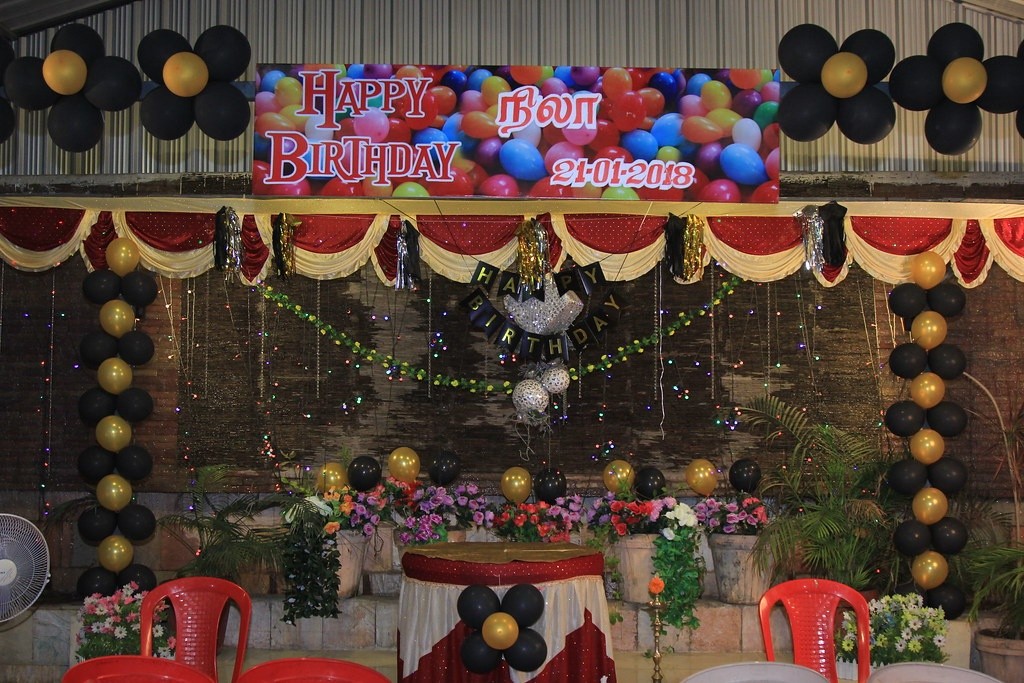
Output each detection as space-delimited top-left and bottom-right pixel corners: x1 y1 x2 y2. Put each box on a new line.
534 468 566 502
500 583 544 629
461 631 502 674
348 456 381 492
483 613 518 650
686 458 718 496
137 25 251 141
777 22 1024 155
729 458 761 494
388 447 419 480
458 584 501 629
76 237 157 601
503 628 548 672
428 450 460 487
254 64 779 206
0 22 141 153
318 462 348 494
886 251 968 620
501 466 533 504
603 460 633 494
634 466 665 501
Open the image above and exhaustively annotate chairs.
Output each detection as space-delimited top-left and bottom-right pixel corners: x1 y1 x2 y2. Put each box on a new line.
61 577 1006 683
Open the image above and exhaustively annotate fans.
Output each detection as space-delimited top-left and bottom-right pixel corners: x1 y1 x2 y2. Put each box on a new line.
0 513 51 624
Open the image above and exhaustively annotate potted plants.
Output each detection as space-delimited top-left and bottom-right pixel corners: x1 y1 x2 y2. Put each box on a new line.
45 461 328 657
960 396 1024 683
724 398 924 647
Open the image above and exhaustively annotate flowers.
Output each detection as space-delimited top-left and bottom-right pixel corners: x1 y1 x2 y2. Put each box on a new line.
321 477 407 542
73 579 176 663
691 495 771 536
387 479 486 548
485 491 582 543
587 482 709 657
833 593 952 665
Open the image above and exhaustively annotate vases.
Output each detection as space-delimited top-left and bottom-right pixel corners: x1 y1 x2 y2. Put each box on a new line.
705 533 776 606
361 526 408 599
610 530 667 605
322 529 364 597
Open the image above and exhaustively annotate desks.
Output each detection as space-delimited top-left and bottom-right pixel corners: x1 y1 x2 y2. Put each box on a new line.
391 538 617 683
